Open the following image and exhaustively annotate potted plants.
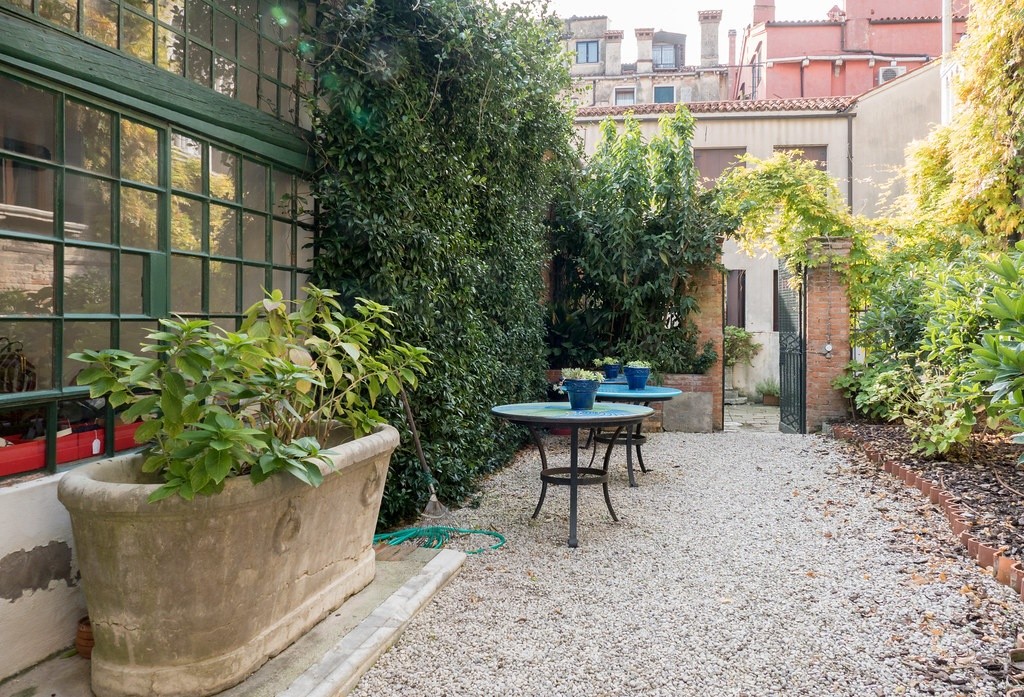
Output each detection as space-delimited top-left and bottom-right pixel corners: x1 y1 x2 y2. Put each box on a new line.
592 357 621 379
623 360 652 390
56 281 435 697
724 324 764 399
560 368 605 410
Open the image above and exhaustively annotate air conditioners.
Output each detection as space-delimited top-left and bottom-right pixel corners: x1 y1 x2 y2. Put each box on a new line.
877 65 907 85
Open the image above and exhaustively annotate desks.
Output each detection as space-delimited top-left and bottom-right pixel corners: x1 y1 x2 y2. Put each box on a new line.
559 373 627 449
490 402 655 547
561 384 682 488
